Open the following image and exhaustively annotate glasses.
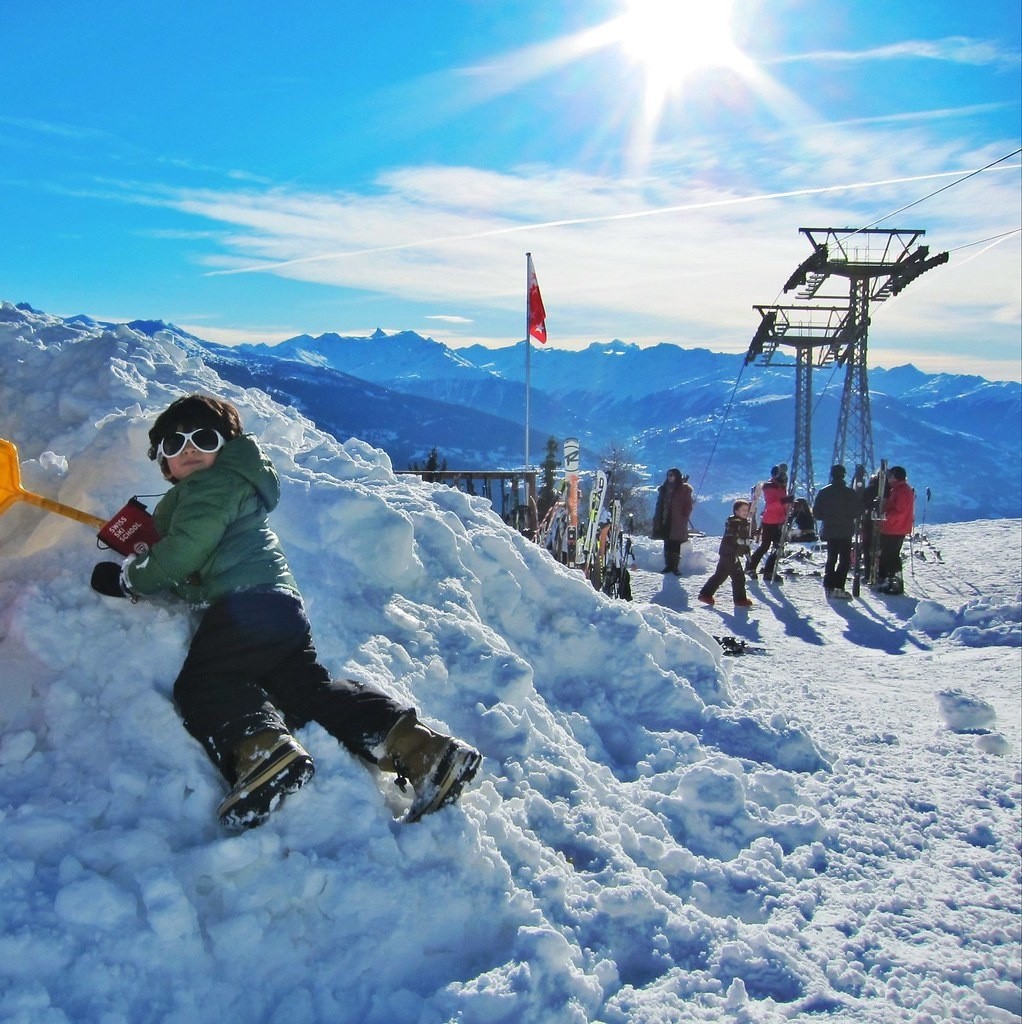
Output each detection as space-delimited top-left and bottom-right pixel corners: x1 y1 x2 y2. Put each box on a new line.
157 427 228 465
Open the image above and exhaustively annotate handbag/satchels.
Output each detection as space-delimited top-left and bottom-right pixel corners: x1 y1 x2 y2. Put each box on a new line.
97 495 160 557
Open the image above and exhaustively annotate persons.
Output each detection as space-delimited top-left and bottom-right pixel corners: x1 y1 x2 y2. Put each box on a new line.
626 513 634 532
862 466 914 595
545 489 582 565
744 463 788 581
651 468 693 576
92 395 482 831
813 464 863 598
698 500 752 607
595 500 633 602
784 498 815 542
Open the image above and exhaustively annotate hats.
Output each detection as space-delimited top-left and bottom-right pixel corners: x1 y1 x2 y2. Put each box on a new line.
888 466 906 480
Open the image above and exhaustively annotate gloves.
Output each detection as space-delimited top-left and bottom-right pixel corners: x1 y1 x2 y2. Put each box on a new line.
90 562 130 598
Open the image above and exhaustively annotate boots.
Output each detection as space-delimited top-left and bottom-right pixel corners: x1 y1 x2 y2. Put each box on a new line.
215 728 315 831
376 708 483 823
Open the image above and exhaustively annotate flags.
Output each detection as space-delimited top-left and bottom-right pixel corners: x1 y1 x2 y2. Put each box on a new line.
528 257 547 343
926 488 931 502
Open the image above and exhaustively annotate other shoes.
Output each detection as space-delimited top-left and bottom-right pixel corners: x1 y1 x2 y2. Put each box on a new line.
698 594 715 605
762 572 783 582
734 599 753 606
823 587 852 599
744 568 758 580
671 566 681 575
661 567 671 574
